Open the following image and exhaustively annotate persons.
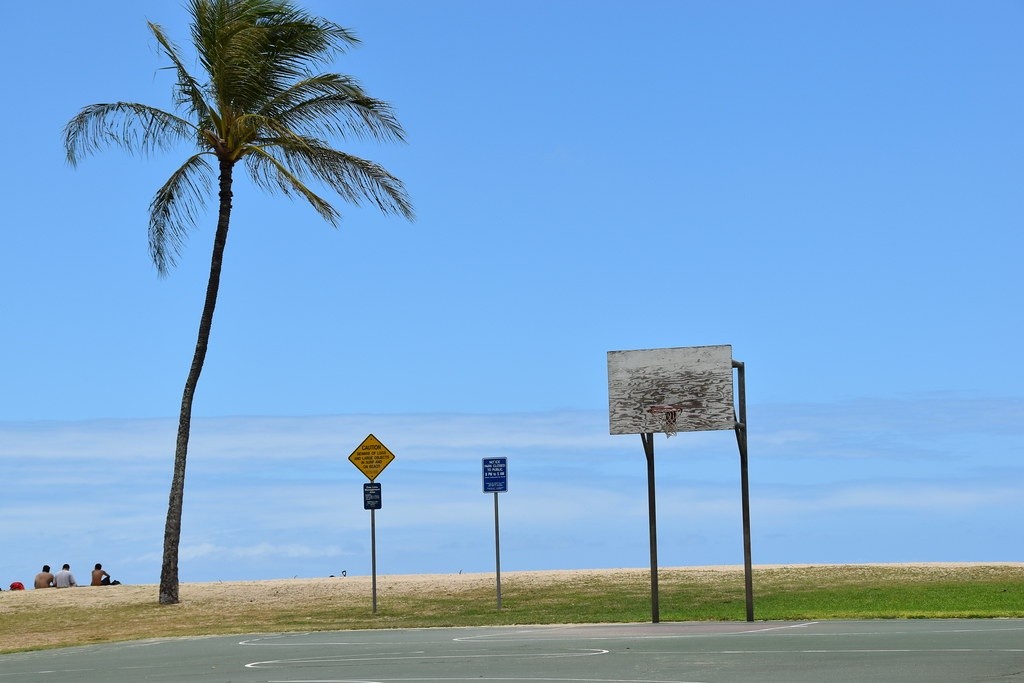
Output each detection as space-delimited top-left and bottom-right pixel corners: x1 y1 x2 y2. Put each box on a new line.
53 564 75 588
90 564 110 586
34 565 54 589
